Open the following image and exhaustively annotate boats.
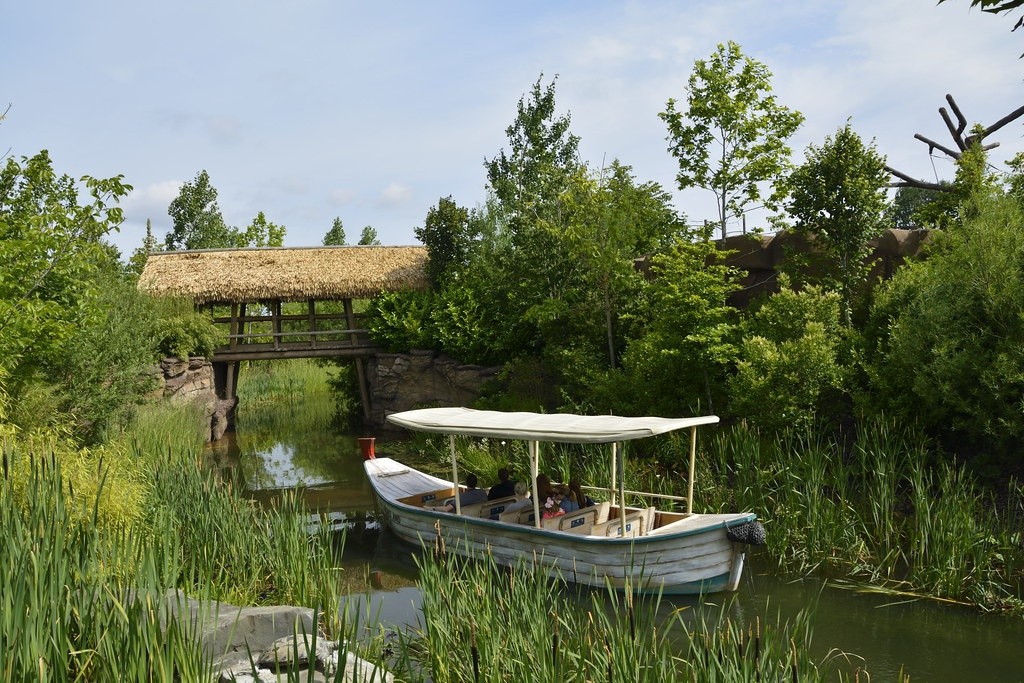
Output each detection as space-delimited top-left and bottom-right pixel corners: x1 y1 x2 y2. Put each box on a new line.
363 407 757 595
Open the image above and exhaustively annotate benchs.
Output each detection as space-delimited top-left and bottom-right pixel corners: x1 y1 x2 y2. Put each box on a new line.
424 486 657 537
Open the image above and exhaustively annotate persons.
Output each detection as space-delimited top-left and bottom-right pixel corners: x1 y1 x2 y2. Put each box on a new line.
541 480 595 527
503 482 532 511
423 474 488 513
530 474 554 499
487 468 516 500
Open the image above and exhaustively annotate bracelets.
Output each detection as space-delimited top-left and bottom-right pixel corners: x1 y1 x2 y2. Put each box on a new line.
433 506 436 510
